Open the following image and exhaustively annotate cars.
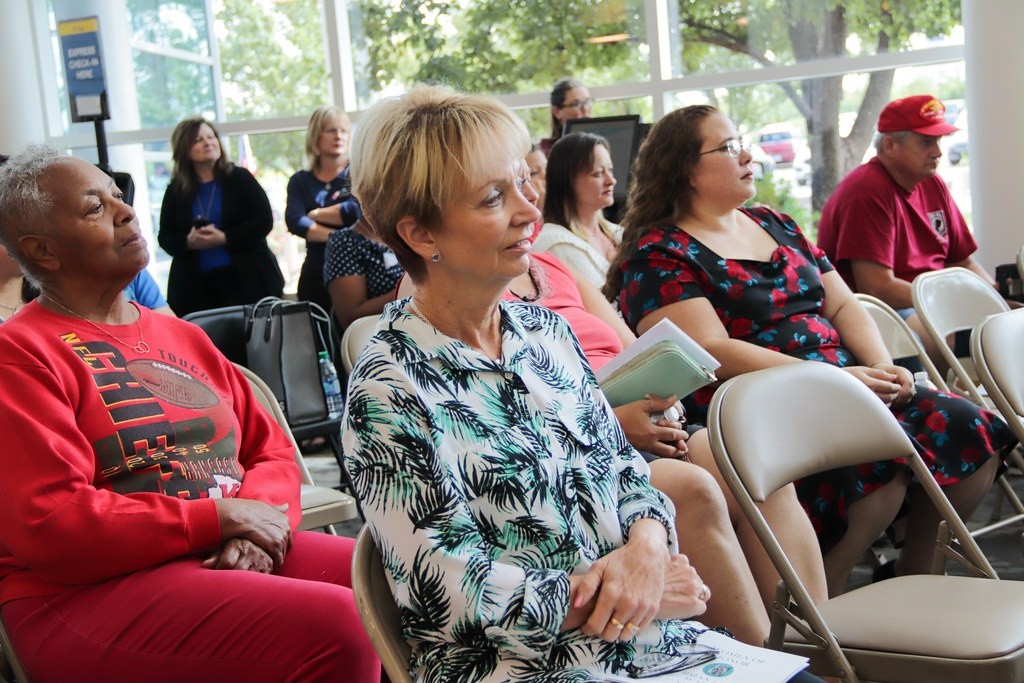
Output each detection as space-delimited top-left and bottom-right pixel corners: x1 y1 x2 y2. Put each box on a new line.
947 105 970 163
760 129 795 163
940 99 960 127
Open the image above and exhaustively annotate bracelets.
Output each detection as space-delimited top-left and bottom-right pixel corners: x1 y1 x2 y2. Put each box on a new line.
870 361 889 368
313 208 320 222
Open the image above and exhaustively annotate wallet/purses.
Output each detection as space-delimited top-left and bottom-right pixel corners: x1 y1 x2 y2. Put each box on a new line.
599 340 718 418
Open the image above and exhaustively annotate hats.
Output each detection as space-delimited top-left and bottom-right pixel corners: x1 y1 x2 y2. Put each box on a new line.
878 95 961 136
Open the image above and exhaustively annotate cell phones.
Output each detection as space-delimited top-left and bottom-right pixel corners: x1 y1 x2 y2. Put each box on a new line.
193 219 208 229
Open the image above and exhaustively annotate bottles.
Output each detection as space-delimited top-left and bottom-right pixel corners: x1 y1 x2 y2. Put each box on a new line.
317 351 343 421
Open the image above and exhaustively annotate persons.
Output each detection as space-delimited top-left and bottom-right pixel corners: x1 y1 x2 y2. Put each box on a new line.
0 81 1017 683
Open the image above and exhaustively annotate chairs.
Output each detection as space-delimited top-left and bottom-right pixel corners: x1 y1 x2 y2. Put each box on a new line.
111 264 1024 683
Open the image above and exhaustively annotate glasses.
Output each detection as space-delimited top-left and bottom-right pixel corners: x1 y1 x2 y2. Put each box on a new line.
699 136 752 157
560 97 594 111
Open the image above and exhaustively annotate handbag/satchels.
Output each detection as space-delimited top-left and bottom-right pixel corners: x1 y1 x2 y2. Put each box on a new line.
243 301 329 428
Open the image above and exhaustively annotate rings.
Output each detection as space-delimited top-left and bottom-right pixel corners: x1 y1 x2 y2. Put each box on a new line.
699 585 707 600
611 617 624 629
626 622 640 634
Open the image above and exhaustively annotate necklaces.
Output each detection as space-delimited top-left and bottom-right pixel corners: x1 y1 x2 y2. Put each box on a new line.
196 183 216 220
410 297 433 327
0 298 22 315
509 268 541 301
43 295 150 354
